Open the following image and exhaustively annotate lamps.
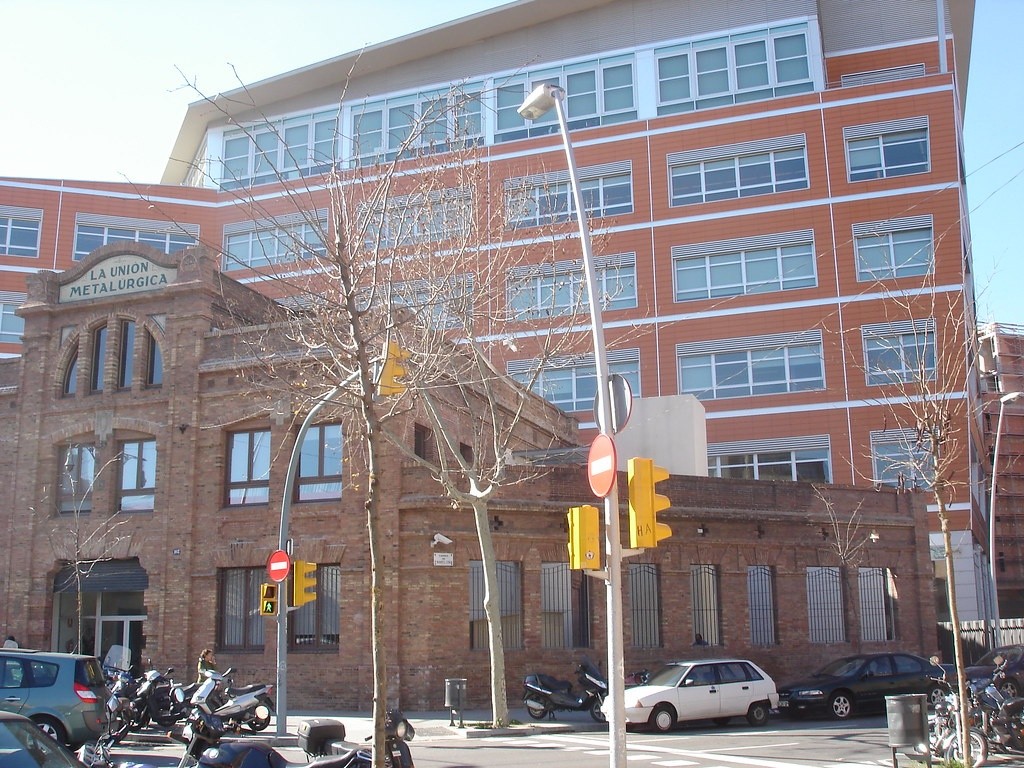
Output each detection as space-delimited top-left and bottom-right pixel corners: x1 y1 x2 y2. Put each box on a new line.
64 431 105 491
430 532 453 548
123 453 147 488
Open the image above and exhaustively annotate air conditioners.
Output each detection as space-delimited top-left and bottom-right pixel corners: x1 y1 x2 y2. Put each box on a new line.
978 378 989 394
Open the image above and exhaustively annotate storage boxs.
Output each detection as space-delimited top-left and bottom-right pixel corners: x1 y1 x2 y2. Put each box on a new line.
298 719 346 754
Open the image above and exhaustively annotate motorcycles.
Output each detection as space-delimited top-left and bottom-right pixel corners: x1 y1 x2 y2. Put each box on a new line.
928 652 1023 768
71 695 416 768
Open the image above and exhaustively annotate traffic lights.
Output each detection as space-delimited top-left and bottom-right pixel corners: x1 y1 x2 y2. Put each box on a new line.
379 340 407 397
629 458 673 549
292 560 319 607
260 584 278 615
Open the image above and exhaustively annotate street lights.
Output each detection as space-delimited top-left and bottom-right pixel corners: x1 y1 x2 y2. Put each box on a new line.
518 83 630 767
988 391 1023 646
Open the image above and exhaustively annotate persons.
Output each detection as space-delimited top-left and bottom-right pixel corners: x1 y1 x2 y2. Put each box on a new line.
693 634 709 646
82 627 109 656
7 635 21 648
196 648 217 684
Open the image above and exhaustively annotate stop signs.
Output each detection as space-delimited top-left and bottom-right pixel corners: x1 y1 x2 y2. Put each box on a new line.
586 436 620 495
268 548 291 582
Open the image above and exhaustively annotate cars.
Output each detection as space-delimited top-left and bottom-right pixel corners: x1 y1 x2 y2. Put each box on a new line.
778 652 957 720
599 656 780 731
0 709 85 768
0 648 111 747
964 644 1023 700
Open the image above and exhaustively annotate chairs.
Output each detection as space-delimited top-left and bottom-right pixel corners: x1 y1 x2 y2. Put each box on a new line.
704 672 714 685
688 672 698 681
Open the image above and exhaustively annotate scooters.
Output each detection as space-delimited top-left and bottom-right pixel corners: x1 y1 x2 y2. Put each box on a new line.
102 646 276 733
524 654 608 724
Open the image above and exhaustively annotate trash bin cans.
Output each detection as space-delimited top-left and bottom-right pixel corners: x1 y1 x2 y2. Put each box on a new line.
884 693 929 747
444 678 467 707
